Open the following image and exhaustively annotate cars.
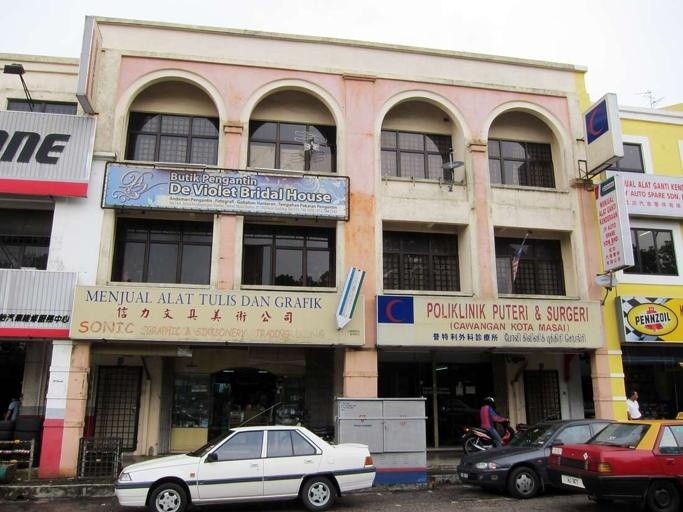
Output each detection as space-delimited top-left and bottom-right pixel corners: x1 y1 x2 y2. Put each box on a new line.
457 412 683 512
115 426 376 512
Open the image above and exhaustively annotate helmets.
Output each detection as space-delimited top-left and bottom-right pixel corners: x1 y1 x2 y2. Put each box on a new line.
483 396 496 403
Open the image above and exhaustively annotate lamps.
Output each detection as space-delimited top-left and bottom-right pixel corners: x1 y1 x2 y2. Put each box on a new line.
3 62 35 112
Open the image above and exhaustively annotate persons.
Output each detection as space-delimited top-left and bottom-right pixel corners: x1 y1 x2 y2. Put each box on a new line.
626 391 643 420
4 396 20 421
480 397 510 447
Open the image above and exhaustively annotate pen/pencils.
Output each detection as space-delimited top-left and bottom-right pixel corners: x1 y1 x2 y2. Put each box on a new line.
335 267 366 331
